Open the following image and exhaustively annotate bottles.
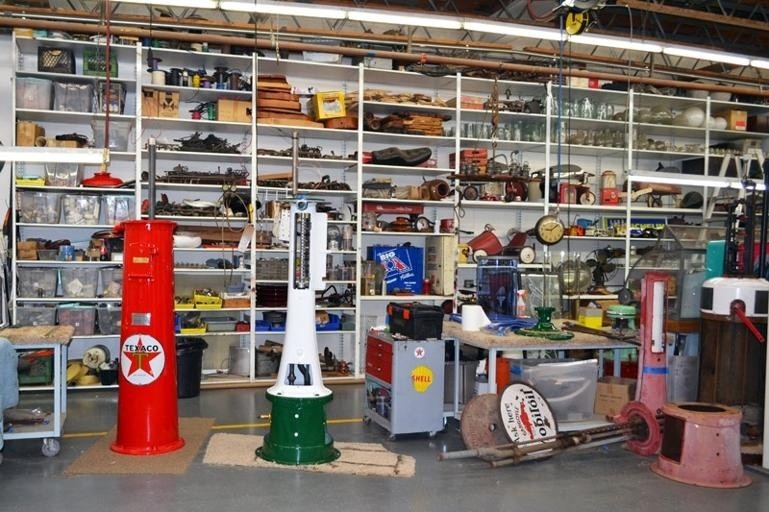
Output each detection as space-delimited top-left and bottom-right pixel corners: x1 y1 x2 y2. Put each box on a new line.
100 239 108 261
421 179 450 199
506 96 624 147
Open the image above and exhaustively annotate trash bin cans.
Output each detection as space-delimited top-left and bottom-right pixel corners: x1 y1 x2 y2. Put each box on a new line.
176 337 208 399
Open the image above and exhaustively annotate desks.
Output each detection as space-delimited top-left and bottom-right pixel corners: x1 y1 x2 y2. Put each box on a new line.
430 316 647 436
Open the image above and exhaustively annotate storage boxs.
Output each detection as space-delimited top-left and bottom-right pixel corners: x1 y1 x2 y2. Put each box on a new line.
81 46 119 78
45 162 83 189
94 267 128 297
8 301 57 329
497 352 604 423
309 88 350 122
59 266 99 301
51 81 92 113
91 302 124 334
16 189 66 225
53 305 96 337
14 76 52 112
58 191 100 226
96 192 137 230
16 264 58 301
33 41 76 75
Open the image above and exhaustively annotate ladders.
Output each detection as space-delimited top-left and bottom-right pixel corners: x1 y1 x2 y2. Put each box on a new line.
689 148 766 272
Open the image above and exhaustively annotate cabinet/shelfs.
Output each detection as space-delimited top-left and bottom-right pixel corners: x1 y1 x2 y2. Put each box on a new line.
2 27 766 438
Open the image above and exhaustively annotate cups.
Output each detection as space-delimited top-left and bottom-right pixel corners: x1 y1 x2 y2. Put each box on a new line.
451 123 506 142
440 218 454 233
